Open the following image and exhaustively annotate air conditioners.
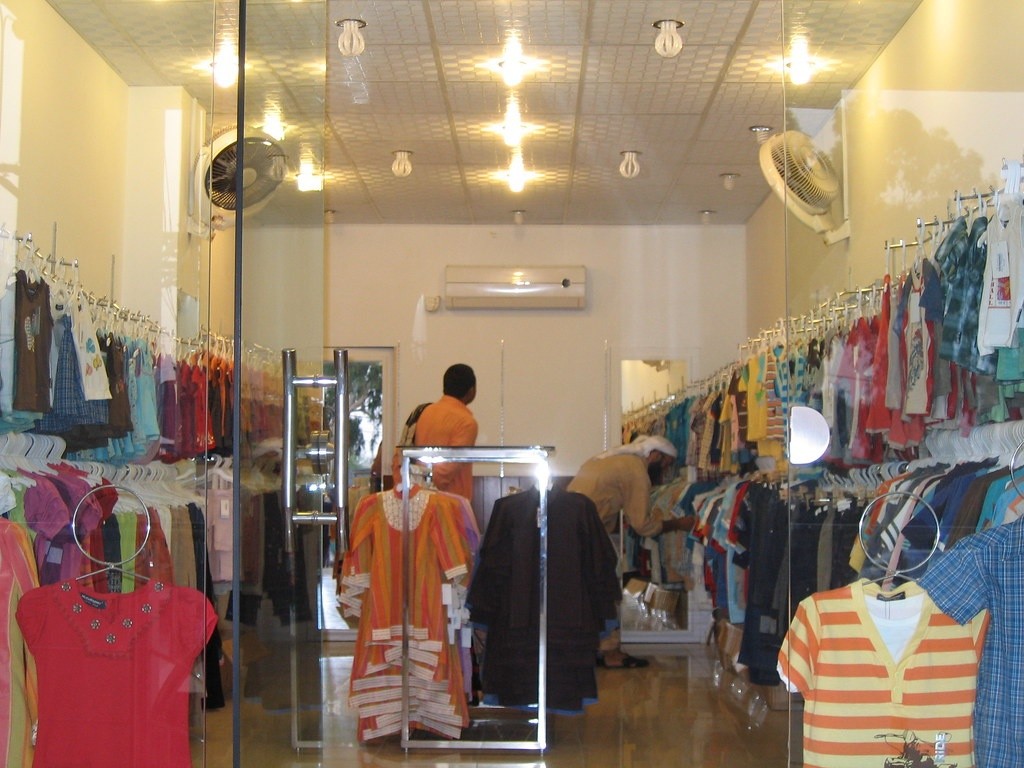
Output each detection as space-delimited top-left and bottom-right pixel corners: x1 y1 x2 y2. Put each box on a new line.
445 264 589 313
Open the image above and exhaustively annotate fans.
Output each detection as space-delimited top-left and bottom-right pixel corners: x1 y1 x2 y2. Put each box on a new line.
187 123 289 240
758 130 850 246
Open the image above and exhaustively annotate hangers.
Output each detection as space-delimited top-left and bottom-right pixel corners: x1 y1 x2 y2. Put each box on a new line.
619 183 1024 516
397 463 431 491
8 231 274 382
0 431 321 519
533 477 555 493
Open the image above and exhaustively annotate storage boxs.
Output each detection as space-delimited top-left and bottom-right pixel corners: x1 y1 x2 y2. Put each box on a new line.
625 579 681 620
716 620 748 676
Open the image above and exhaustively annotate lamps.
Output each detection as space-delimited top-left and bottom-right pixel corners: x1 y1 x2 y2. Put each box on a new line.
699 210 718 225
333 17 369 58
390 149 415 178
719 172 741 191
653 17 688 59
618 149 644 178
749 125 774 145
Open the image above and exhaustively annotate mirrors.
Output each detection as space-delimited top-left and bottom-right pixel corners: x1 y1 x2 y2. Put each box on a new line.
599 348 705 645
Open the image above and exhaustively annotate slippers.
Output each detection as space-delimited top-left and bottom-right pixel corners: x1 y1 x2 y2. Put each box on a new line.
604 653 650 667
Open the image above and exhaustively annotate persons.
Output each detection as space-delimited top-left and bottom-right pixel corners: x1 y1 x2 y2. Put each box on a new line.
390 364 479 500
568 434 695 669
